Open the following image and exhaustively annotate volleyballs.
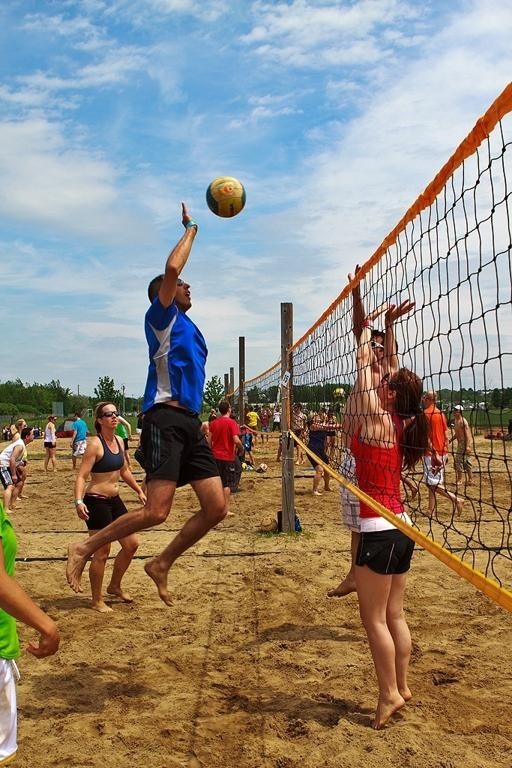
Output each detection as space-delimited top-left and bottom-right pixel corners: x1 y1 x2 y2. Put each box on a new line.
206 176 245 217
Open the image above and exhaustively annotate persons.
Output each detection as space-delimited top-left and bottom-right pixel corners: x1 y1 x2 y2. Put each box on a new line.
63 200 228 607
113 415 133 469
1 418 44 515
445 403 475 486
1 496 64 768
351 297 432 732
195 391 350 512
72 400 149 615
70 410 89 471
43 415 58 473
320 263 401 599
419 389 465 520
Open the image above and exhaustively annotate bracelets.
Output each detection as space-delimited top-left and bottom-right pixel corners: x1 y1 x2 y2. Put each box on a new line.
362 319 375 329
74 498 84 507
184 219 198 231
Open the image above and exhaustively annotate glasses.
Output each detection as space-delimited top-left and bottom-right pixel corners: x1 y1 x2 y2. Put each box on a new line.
175 278 184 286
100 411 120 417
380 371 395 389
369 339 385 350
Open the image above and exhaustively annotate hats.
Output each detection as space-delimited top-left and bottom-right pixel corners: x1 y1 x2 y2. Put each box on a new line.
452 402 464 410
